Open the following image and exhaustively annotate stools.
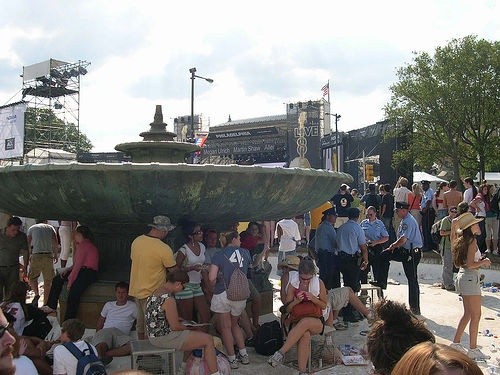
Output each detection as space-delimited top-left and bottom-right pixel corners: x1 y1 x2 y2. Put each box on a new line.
129 339 176 375
360 284 381 314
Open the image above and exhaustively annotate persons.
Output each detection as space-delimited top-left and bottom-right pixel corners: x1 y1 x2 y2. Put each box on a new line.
40 225 100 324
0 177 500 375
209 230 252 369
144 266 221 374
267 256 327 374
452 211 492 360
364 296 436 375
390 340 484 375
368 200 423 319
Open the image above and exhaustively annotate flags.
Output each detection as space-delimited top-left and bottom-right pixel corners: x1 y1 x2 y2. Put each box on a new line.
194 136 207 154
320 83 329 97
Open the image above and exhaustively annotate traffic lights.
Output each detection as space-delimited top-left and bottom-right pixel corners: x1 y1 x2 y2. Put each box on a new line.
325 112 341 172
365 165 374 181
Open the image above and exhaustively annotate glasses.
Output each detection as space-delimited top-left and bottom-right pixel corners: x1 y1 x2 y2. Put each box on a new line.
0 322 10 338
190 231 203 235
299 274 313 281
181 283 185 290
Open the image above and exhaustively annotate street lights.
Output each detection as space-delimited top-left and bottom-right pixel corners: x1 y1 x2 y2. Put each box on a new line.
189 68 213 142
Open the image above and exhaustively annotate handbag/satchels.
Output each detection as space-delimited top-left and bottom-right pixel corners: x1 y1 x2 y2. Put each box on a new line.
283 297 324 326
316 340 343 365
185 346 232 375
339 293 372 322
3 303 63 358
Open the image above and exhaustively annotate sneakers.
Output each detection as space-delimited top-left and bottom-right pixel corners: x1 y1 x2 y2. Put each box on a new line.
230 352 250 369
267 350 284 368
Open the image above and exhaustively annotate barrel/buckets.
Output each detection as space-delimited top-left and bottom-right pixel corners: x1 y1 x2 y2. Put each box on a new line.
186 349 232 375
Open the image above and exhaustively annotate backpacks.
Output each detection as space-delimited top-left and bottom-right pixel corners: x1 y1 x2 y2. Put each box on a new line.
431 217 452 245
221 248 251 301
255 320 286 356
61 340 108 375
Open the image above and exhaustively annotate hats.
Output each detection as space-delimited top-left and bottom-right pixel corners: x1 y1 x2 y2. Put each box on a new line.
278 255 300 271
455 212 484 236
420 179 432 185
147 216 177 231
393 201 410 211
322 207 337 216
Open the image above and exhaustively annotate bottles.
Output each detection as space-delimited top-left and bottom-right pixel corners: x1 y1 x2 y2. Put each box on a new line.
489 288 497 292
367 271 372 286
344 343 352 355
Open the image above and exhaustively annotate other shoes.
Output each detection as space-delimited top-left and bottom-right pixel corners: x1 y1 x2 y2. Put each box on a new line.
334 321 350 329
32 295 40 305
40 305 56 313
234 336 256 350
493 250 499 255
369 280 386 289
485 248 491 253
441 283 455 291
448 341 491 359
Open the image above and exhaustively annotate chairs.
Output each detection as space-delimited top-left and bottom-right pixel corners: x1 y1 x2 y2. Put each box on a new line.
280 304 336 374
